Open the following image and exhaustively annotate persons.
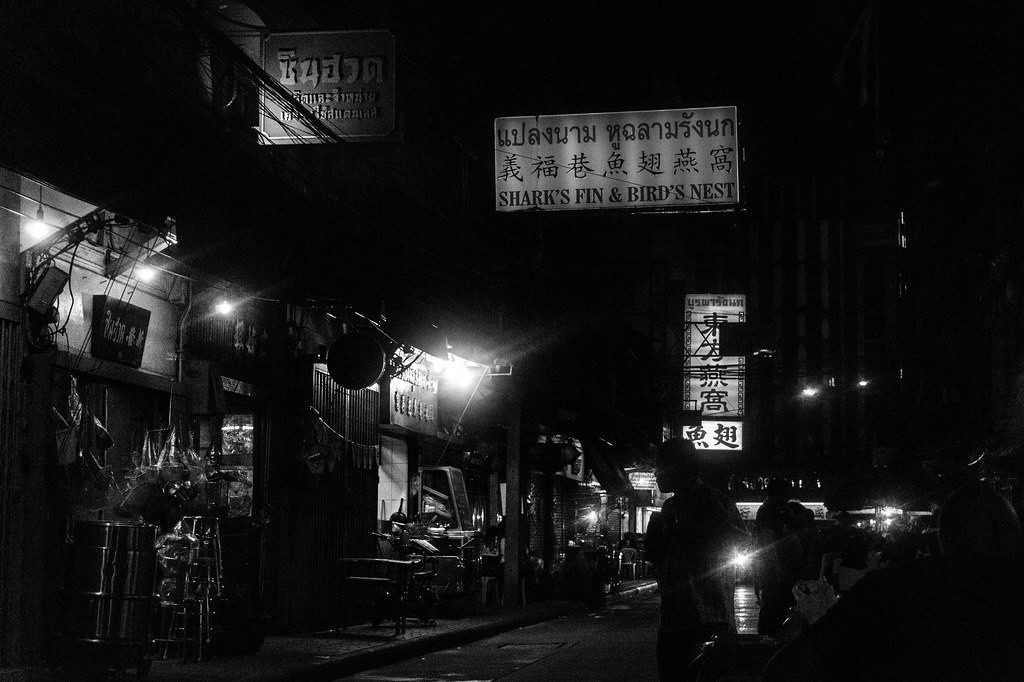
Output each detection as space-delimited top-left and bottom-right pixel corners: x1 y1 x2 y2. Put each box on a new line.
588 536 610 618
642 437 747 682
616 532 643 579
748 469 1020 639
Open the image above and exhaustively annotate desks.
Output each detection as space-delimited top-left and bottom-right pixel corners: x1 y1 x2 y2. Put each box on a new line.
342 555 421 634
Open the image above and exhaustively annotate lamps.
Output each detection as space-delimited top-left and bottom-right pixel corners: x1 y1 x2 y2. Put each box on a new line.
390 500 407 526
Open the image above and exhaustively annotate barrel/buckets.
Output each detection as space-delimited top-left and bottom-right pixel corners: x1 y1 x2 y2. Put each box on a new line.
62 518 159 674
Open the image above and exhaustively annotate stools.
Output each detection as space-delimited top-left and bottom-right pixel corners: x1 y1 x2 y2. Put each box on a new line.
153 513 227 663
